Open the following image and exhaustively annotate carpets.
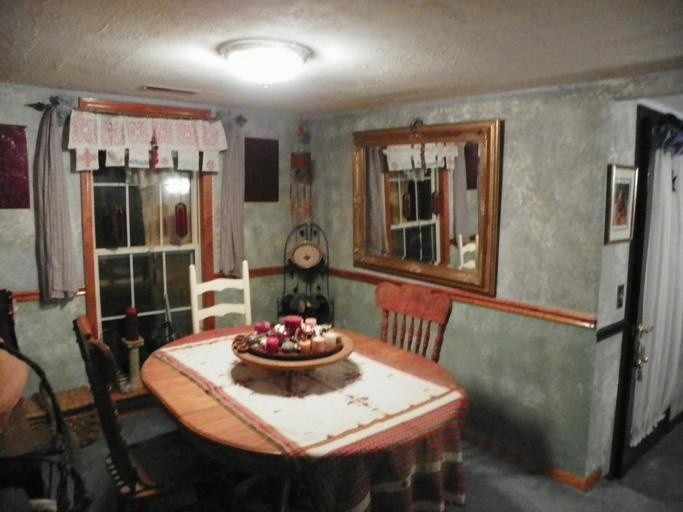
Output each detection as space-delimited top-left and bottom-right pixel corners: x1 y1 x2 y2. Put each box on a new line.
27 395 683 512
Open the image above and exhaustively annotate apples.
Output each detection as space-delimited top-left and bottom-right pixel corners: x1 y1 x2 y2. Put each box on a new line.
267 337 280 352
254 321 271 334
283 315 303 336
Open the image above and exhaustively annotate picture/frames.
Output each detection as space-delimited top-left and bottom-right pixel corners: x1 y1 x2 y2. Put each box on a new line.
606 164 639 244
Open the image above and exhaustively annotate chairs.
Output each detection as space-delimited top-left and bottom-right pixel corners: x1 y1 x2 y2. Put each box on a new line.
72 318 209 512
374 284 452 362
458 235 478 271
189 261 251 333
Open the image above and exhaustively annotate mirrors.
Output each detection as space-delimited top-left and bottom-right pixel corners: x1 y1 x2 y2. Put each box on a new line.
352 119 502 297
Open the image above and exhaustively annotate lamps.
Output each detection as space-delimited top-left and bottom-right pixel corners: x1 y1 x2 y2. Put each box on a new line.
218 38 314 88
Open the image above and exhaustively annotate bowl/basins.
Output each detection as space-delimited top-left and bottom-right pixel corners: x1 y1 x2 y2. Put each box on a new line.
231 328 352 372
